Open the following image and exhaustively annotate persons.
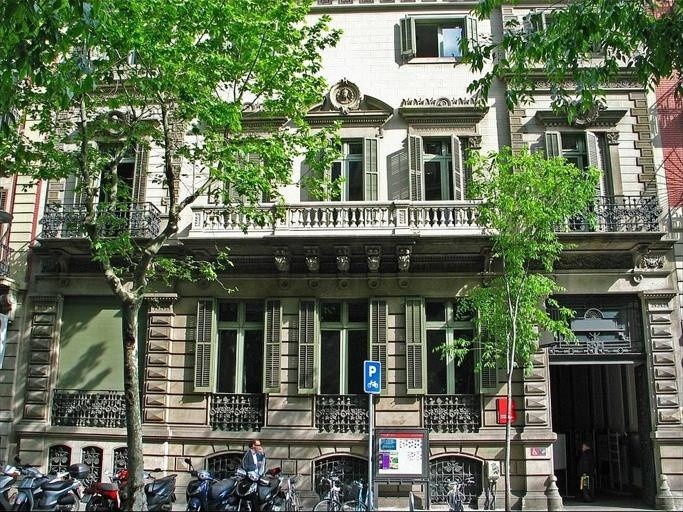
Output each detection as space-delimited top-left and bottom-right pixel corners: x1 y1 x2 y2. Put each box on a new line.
576 442 597 502
238 439 265 480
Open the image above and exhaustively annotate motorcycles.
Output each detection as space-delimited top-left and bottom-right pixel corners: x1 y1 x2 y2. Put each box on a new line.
0 456 284 512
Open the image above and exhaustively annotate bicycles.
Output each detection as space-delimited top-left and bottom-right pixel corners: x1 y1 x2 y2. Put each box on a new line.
442 476 475 512
313 475 376 512
